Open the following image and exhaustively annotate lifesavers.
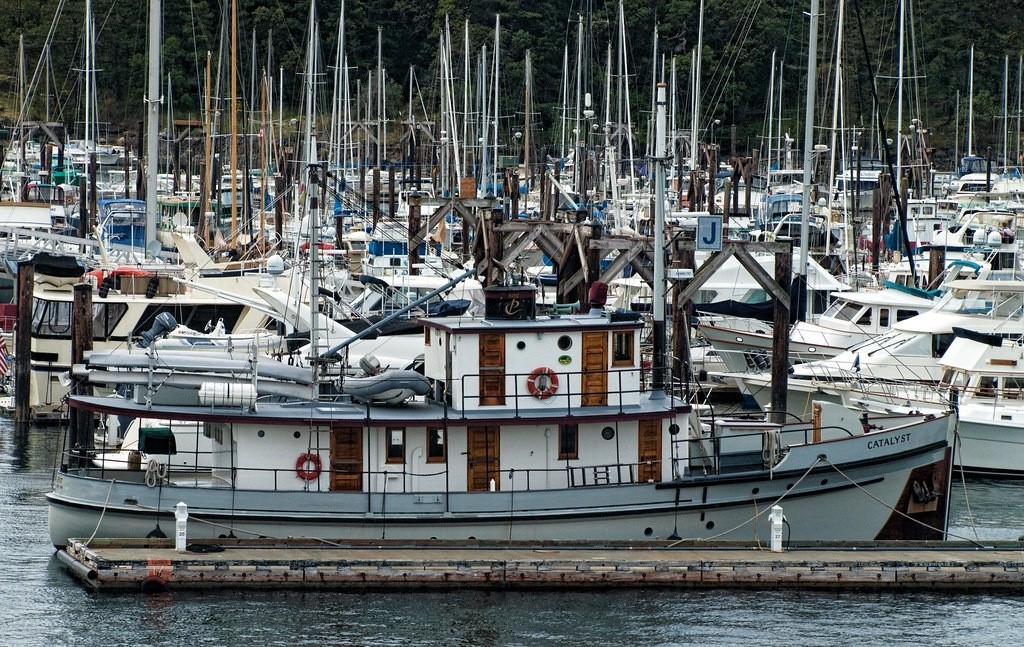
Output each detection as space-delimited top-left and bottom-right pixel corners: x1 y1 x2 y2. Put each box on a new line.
297 454 322 479
527 367 560 399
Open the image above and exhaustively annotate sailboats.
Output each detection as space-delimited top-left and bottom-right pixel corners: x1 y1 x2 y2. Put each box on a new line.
0 0 1024 544
